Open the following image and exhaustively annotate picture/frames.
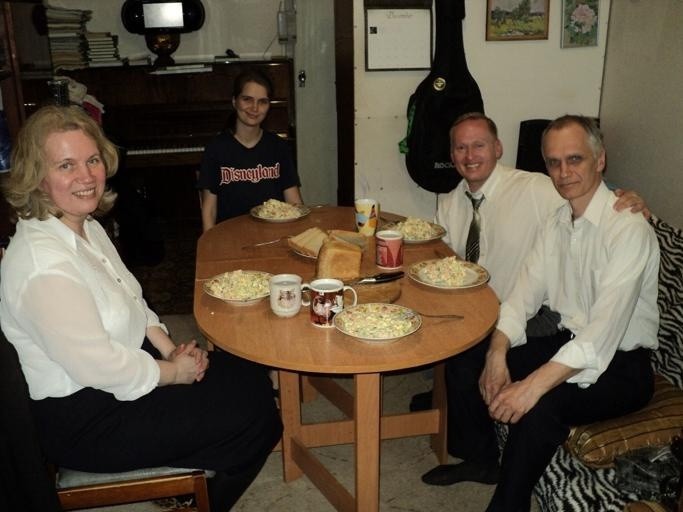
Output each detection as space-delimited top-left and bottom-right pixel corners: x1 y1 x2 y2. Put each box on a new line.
561 0 601 48
485 0 549 41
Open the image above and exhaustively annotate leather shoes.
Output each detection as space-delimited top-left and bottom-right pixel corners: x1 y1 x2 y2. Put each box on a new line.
421 464 502 485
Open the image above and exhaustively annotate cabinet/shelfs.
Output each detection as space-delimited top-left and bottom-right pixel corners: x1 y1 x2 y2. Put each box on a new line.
0 0 55 265
55 57 297 315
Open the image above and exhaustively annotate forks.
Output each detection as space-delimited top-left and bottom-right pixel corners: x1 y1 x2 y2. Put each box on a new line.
400 308 465 322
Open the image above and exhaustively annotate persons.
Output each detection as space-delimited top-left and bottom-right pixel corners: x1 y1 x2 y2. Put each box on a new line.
410 111 650 456
198 74 305 418
0 102 286 510
417 116 663 511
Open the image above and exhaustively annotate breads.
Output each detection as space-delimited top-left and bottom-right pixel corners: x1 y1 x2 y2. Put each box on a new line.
287 228 366 280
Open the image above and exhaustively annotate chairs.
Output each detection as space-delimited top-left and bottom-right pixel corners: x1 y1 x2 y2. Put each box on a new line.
0 328 217 512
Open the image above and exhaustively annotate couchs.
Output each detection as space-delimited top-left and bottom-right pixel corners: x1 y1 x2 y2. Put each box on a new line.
495 211 683 512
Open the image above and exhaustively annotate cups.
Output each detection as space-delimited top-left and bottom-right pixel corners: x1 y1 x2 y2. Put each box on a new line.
268 272 311 318
352 198 381 237
374 231 406 271
308 279 358 331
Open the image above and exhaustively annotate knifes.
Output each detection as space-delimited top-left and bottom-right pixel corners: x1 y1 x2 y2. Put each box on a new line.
301 271 406 293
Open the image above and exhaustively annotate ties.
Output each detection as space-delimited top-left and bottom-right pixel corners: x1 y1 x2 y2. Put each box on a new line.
465 192 484 262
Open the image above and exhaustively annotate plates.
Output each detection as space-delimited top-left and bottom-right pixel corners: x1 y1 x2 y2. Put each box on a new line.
334 302 423 344
290 248 366 260
407 256 492 290
379 218 448 246
250 203 313 223
202 268 276 305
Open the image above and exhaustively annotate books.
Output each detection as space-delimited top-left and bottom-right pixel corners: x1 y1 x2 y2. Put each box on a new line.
47 4 122 72
128 53 151 66
